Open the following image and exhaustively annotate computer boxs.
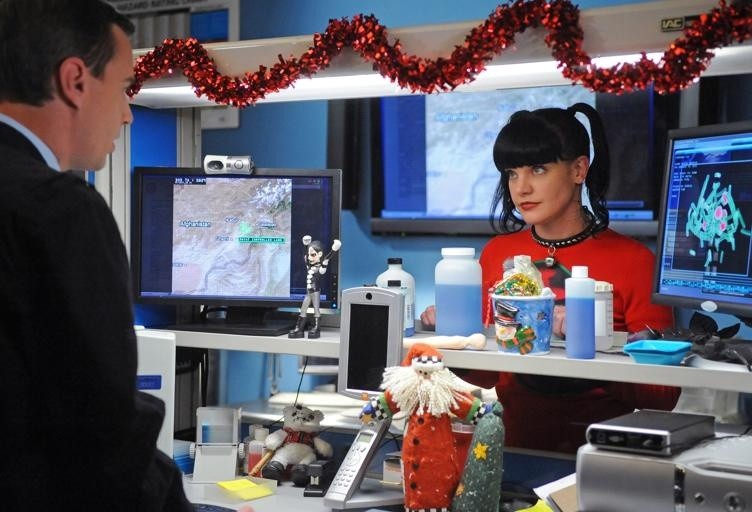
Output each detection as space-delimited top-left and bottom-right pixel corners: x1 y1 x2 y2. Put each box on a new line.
576 422 752 512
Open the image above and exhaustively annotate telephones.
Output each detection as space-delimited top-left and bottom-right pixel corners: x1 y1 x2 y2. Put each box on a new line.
323 419 405 510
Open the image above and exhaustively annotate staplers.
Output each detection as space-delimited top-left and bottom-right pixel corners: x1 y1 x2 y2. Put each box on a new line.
304 460 339 497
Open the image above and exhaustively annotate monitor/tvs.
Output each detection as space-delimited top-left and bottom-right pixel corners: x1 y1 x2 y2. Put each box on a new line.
358 79 701 240
130 166 343 337
650 119 752 367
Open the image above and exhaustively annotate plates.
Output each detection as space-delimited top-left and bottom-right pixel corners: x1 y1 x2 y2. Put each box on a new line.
624 339 694 365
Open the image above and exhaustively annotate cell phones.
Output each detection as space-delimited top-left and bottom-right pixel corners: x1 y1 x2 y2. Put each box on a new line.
326 420 387 494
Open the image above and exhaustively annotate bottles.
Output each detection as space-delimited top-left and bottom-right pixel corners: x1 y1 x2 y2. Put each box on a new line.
565 264 614 358
377 259 416 337
243 424 269 477
434 245 482 339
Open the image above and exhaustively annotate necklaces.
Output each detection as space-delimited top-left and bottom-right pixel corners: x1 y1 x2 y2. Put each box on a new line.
530 211 597 276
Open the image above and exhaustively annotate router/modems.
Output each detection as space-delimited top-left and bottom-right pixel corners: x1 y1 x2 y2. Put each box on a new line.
585 408 716 459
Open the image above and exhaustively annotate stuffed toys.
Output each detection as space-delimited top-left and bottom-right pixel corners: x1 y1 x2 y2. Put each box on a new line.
262 402 333 486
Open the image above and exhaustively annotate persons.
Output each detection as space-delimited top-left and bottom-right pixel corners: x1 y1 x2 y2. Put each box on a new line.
420 103 673 454
359 343 504 512
287 235 341 338
0 0 196 512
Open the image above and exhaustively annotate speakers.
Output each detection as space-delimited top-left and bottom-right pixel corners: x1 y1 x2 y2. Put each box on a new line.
335 285 407 404
